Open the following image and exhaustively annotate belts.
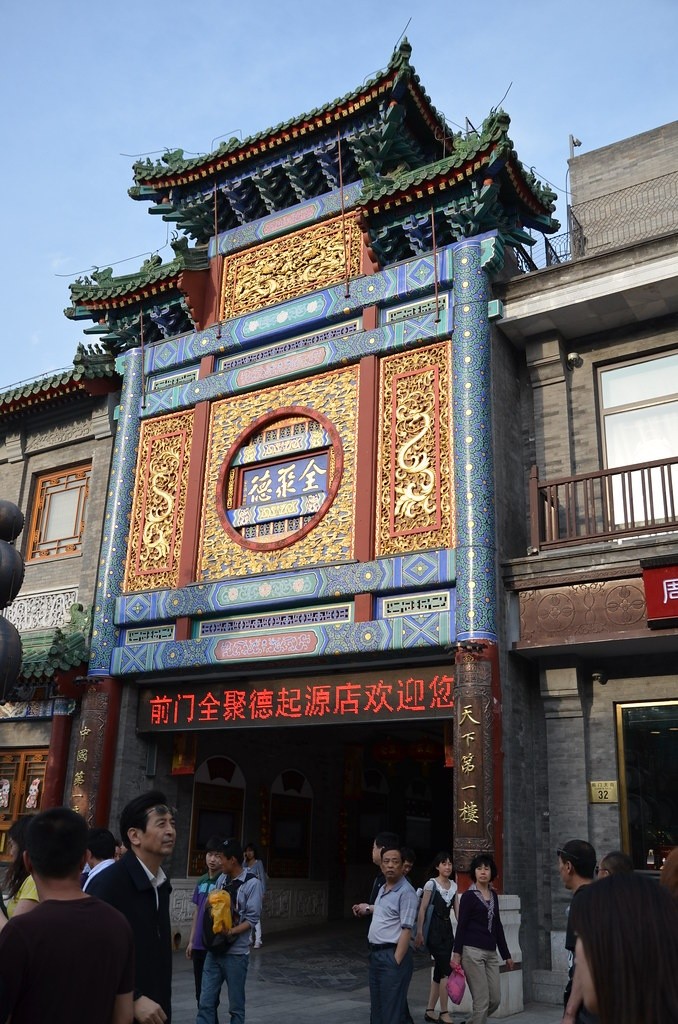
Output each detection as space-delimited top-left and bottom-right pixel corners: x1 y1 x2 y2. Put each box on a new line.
368 942 396 951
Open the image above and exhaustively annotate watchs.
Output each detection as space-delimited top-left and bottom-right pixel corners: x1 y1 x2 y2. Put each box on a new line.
366 905 371 914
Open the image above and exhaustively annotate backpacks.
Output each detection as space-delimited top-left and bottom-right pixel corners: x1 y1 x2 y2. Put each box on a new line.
204 873 258 952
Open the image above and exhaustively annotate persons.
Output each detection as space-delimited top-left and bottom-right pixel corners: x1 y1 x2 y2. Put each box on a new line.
557 840 678 1024
185 837 270 1024
0 815 41 933
414 853 460 1024
0 807 140 1024
352 834 422 1024
85 790 178 1024
451 853 514 1024
78 827 129 900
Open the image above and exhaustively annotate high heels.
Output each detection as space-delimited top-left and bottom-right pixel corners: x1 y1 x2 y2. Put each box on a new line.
424 1008 454 1024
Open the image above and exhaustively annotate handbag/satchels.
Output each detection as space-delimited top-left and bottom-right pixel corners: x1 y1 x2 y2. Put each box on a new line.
410 879 436 953
446 962 466 1005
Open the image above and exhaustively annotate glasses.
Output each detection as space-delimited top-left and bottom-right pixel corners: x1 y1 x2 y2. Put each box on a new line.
140 804 178 820
557 848 579 859
595 866 610 875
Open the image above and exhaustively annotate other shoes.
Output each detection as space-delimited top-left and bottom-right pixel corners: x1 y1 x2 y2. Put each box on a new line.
254 941 262 948
